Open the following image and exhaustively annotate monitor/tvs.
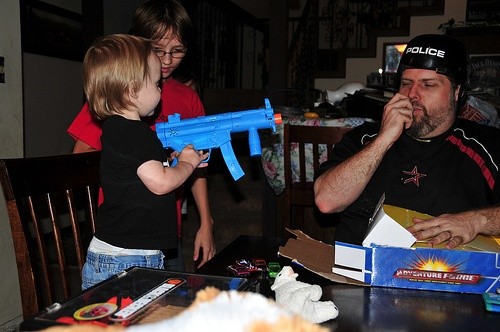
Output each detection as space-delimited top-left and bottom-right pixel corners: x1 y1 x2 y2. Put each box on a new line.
383 42 407 74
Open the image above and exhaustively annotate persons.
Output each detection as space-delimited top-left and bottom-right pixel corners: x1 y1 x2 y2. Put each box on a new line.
65 0 217 300
312 33 500 250
81 34 209 294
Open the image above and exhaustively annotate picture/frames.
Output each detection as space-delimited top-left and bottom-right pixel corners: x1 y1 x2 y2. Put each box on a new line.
20 0 104 62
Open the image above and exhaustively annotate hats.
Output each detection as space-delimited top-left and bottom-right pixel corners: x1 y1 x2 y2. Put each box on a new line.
398 34 468 83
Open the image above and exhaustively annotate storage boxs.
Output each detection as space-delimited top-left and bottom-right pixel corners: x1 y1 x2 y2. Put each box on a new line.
279 204 500 295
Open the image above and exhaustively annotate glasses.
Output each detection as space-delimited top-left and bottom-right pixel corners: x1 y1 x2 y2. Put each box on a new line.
154 49 186 58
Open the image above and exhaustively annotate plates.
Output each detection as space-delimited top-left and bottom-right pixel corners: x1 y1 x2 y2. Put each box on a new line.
73 302 118 320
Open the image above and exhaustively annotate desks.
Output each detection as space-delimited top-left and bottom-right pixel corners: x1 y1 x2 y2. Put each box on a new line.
13 235 500 332
259 116 372 195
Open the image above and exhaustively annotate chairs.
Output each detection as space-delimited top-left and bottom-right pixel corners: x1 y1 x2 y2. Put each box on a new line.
0 149 103 319
284 124 352 245
